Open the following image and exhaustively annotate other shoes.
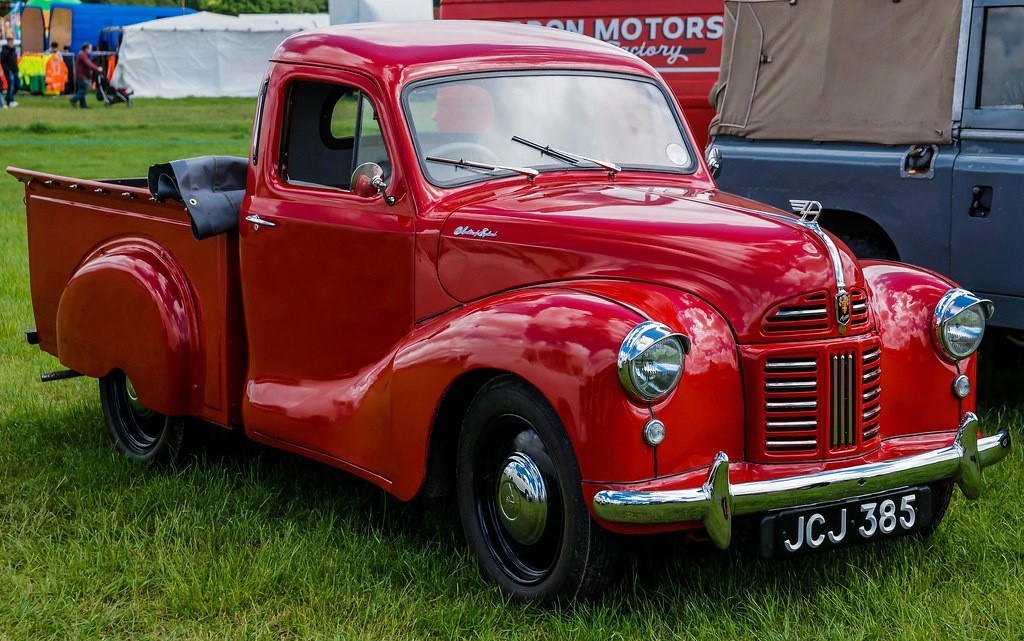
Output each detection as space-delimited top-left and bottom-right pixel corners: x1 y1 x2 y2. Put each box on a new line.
81 106 89 109
9 100 19 108
1 105 7 109
70 98 78 109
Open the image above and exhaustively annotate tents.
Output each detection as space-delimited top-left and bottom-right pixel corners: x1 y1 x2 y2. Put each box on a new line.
110 10 330 97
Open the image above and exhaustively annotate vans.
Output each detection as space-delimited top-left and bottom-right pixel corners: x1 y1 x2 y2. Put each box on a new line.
428 0 726 165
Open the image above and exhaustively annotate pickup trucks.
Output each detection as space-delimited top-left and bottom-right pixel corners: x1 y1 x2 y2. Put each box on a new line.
697 0 1024 373
4 18 1013 608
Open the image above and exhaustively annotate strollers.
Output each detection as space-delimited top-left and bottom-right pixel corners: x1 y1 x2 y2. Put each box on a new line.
92 71 134 109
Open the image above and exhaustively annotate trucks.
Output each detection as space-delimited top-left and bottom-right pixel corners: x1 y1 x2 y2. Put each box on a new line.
17 2 195 70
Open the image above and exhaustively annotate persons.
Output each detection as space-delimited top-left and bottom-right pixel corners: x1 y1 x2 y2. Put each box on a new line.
61 46 71 53
0 38 21 109
68 43 103 108
45 42 63 61
